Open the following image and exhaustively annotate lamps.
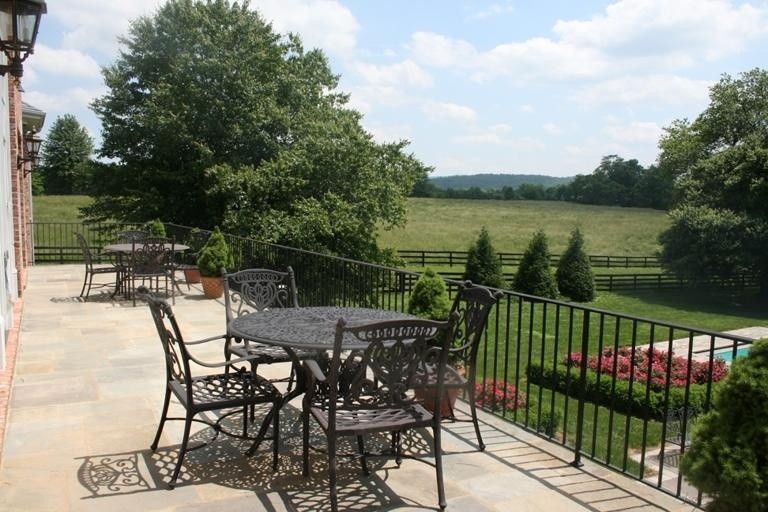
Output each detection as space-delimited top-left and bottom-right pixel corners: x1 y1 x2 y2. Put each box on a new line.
0 0 46 78
17 126 42 176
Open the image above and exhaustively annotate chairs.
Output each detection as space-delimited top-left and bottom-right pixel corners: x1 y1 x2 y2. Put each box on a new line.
412 281 502 451
73 229 188 306
144 295 282 491
223 267 322 425
303 311 458 511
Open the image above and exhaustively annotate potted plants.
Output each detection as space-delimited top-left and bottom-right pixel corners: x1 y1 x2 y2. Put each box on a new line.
407 270 468 420
183 224 227 298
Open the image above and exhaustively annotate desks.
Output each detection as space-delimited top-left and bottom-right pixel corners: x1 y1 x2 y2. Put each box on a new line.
229 304 438 456
